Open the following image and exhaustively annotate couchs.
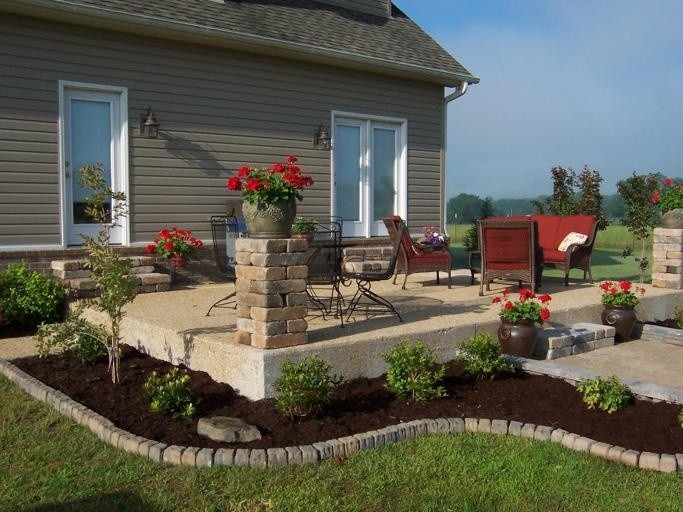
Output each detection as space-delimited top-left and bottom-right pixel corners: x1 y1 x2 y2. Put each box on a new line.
527 214 601 286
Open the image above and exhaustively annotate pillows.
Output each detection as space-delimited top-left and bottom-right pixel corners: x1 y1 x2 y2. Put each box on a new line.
558 232 588 252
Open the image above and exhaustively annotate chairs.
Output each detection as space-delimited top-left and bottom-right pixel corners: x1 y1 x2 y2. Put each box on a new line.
384 215 452 289
206 216 239 315
479 215 544 296
306 216 407 327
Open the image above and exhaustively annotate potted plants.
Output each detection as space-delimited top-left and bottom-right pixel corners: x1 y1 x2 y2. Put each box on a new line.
292 217 319 243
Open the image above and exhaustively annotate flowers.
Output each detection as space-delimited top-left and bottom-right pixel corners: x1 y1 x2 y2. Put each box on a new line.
421 225 450 253
492 280 645 329
146 224 206 260
651 177 683 211
227 155 314 216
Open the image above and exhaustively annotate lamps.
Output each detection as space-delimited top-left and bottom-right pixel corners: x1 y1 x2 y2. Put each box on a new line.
139 107 160 138
312 123 332 150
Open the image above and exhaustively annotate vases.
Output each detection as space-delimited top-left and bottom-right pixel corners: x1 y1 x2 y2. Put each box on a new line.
601 305 637 341
659 208 683 229
498 319 538 358
169 252 185 267
241 199 295 239
